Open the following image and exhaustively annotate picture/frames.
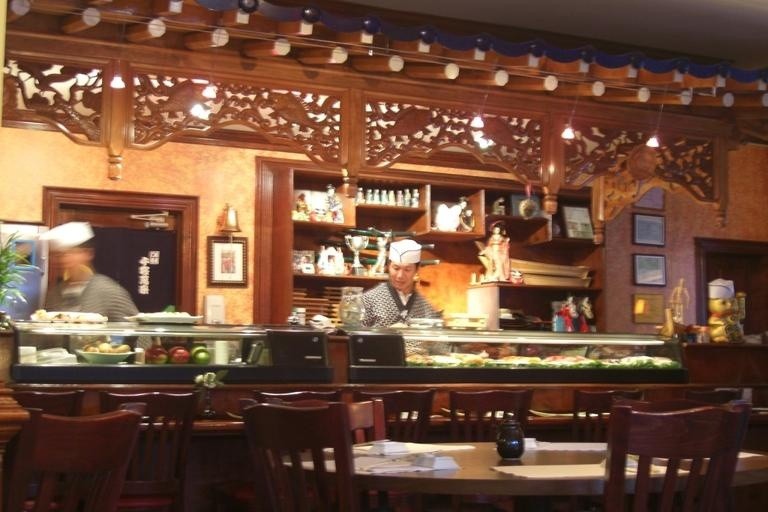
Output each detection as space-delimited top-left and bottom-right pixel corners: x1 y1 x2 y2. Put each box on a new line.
559 205 595 243
205 235 249 291
631 252 668 289
634 187 667 214
631 211 667 250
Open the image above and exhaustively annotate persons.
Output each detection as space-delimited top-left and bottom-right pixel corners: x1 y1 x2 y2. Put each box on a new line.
669 277 691 324
38 221 140 323
348 239 436 380
476 226 510 281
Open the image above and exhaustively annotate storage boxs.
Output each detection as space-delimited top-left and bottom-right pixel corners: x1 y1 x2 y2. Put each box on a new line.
2 266 46 322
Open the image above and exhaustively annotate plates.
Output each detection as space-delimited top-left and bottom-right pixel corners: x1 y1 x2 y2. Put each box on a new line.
124 314 203 325
76 349 135 363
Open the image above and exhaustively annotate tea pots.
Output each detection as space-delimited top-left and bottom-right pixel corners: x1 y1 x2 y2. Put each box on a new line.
494 420 525 460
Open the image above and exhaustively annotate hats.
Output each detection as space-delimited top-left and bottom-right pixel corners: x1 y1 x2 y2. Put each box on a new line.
388 240 422 264
39 221 95 252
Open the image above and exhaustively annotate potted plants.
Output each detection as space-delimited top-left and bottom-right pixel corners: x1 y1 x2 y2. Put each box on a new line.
0 233 34 386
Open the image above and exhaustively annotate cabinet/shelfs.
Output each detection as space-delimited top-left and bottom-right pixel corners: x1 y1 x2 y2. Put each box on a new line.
253 154 605 335
342 327 691 430
10 319 342 445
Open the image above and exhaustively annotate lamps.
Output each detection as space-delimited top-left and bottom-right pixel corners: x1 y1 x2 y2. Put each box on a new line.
217 204 242 243
644 83 673 151
110 18 127 90
201 24 221 101
469 52 503 131
560 64 589 142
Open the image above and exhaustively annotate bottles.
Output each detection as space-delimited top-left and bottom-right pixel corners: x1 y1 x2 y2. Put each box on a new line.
356 188 419 207
